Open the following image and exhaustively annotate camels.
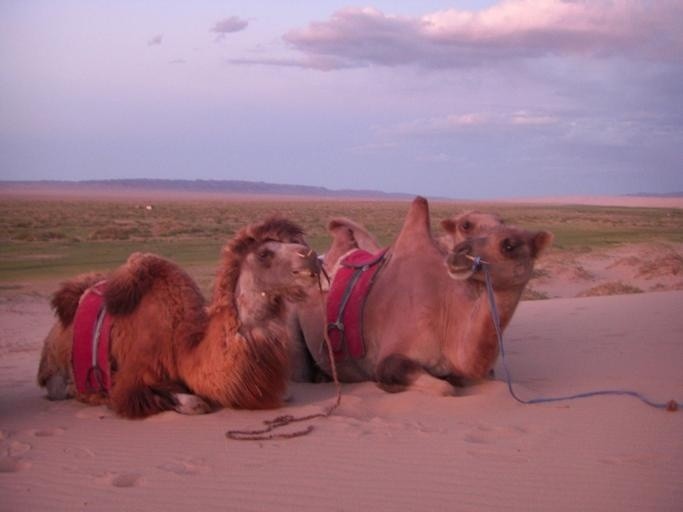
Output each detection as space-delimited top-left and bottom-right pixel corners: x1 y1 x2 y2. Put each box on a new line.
325 209 507 253
35 214 323 420
287 196 555 397
